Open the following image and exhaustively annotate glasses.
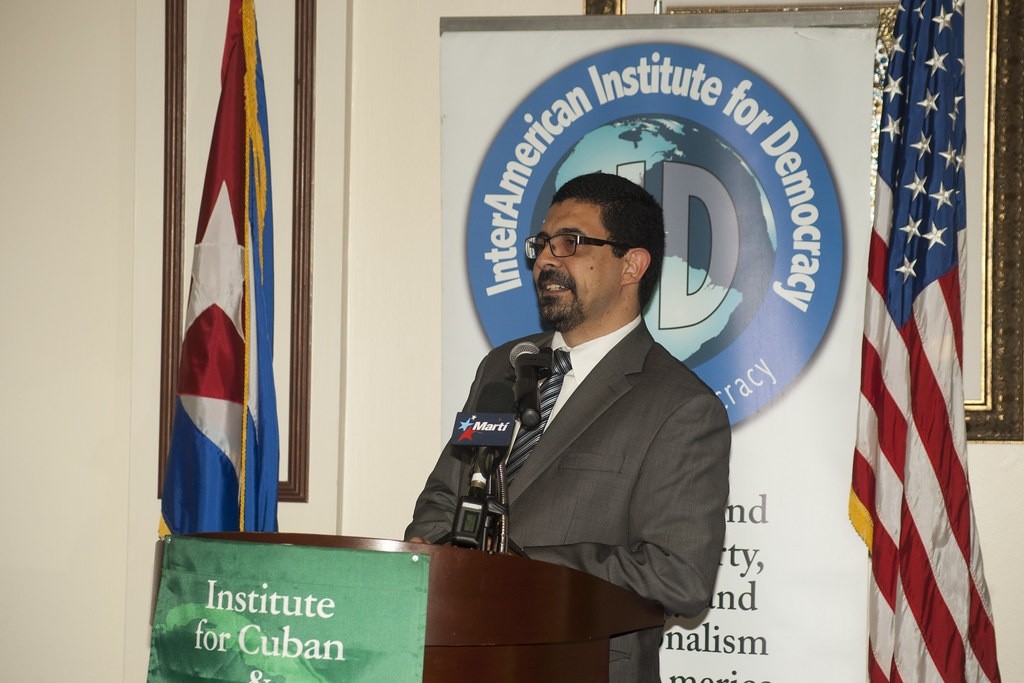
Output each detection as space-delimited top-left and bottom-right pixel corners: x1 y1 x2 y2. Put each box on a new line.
523 233 635 259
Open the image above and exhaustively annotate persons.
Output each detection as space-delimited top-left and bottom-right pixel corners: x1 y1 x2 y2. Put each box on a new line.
401 171 729 617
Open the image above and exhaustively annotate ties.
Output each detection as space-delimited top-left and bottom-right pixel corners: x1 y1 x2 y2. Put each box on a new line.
483 347 575 509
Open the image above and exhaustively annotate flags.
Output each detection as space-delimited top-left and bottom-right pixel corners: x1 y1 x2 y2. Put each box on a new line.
846 0 1003 679
155 2 278 532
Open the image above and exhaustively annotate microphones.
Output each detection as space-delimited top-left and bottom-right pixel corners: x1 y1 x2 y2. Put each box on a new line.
460 382 516 500
509 341 541 429
